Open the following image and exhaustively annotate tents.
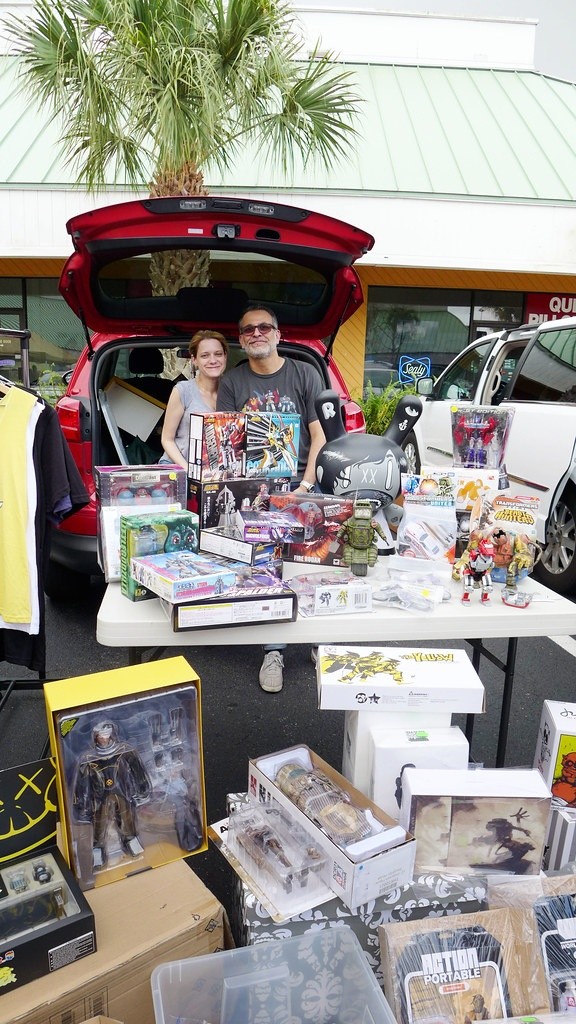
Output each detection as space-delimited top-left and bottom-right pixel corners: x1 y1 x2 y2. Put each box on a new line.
0 332 78 364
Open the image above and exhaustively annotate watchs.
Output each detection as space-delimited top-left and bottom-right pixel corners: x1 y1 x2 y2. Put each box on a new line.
300 481 316 493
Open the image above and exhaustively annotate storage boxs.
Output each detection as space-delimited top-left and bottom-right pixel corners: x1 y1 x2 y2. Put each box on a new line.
0 408 576 1024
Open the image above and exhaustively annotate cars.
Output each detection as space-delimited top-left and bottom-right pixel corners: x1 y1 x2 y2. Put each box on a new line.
362 361 447 404
33 194 376 609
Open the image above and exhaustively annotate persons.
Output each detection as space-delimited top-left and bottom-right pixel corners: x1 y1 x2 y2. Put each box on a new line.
452 527 533 608
158 330 227 471
216 306 332 692
77 724 150 868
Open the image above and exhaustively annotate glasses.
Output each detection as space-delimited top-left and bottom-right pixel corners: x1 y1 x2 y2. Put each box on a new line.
240 323 277 335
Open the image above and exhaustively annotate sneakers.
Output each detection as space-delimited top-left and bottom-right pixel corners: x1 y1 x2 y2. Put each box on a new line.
311 647 319 663
259 651 285 691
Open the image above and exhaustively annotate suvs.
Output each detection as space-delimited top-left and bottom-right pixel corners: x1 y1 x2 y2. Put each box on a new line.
393 316 576 586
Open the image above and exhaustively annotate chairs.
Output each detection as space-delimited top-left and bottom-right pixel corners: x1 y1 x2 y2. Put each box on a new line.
118 348 173 401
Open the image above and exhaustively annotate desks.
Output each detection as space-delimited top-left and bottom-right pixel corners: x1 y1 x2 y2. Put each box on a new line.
98 559 576 768
